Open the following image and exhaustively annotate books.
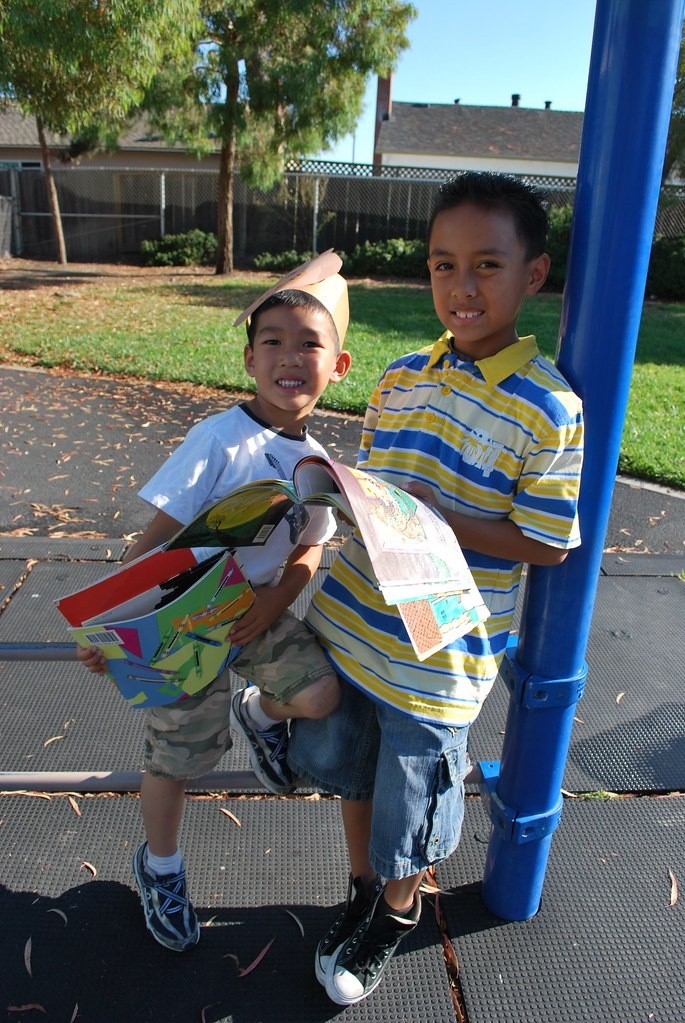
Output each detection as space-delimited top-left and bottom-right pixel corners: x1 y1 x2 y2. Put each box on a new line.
52 453 493 710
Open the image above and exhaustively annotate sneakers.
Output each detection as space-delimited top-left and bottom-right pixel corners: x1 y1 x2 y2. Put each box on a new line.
131 839 201 950
229 685 300 795
316 872 384 986
325 882 421 1008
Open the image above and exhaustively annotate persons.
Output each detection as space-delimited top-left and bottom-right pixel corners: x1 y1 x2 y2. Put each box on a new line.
76 247 353 955
284 171 585 1008
